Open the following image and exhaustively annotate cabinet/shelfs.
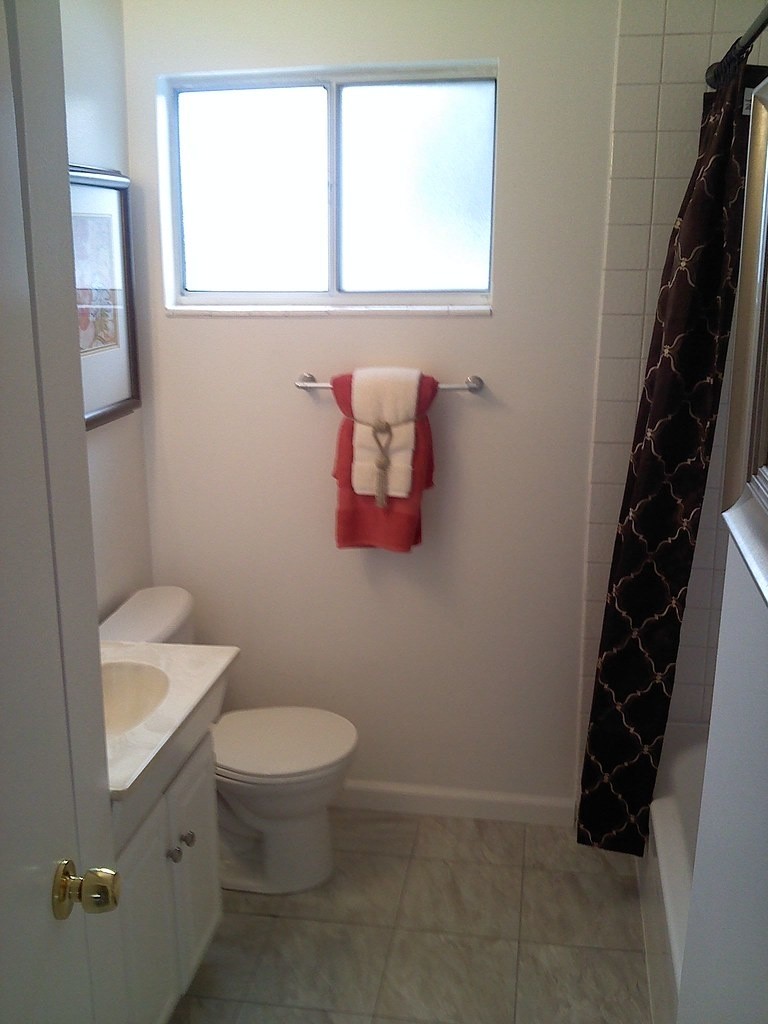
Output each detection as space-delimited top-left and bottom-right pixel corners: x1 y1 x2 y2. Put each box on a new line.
96 637 243 1022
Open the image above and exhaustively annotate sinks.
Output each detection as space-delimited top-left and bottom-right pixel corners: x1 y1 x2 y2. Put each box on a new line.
93 630 241 803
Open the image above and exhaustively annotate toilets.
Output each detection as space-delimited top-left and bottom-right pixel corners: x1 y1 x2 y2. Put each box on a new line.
90 584 366 896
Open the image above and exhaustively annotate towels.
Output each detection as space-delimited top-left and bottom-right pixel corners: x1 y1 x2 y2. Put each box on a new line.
351 364 424 500
328 372 440 554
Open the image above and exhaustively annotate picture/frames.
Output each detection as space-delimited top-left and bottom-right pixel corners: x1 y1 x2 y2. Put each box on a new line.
64 161 142 442
